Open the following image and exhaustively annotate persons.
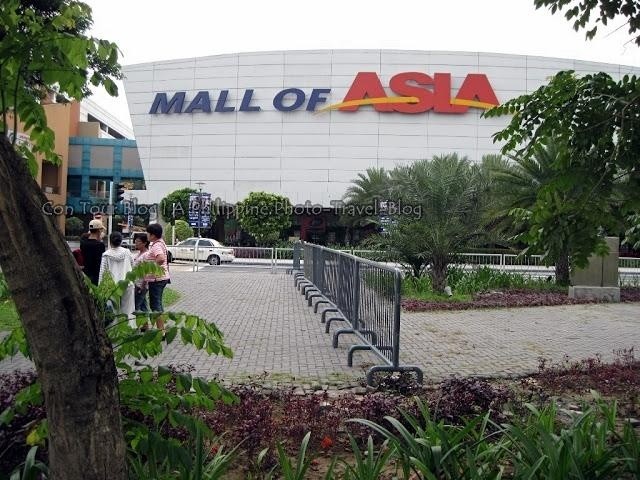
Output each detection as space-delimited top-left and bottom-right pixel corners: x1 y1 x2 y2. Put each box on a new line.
79 220 107 287
98 230 140 331
131 233 150 332
143 222 171 341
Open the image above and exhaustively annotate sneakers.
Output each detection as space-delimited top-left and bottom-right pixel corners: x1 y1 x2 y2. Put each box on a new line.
161 330 170 342
140 327 147 332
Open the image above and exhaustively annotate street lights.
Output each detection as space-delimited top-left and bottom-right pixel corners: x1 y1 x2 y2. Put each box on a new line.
189 180 213 237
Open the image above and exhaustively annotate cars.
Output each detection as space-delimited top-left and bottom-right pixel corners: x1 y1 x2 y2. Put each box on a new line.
121 231 148 249
165 237 236 265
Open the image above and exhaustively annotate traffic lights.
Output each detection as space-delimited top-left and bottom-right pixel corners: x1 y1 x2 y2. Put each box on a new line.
114 184 124 203
169 217 176 226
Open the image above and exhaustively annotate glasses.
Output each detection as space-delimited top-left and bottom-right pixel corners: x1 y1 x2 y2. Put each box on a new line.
134 239 143 243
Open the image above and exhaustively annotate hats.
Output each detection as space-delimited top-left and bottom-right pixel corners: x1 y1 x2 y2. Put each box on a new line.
88 219 107 232
